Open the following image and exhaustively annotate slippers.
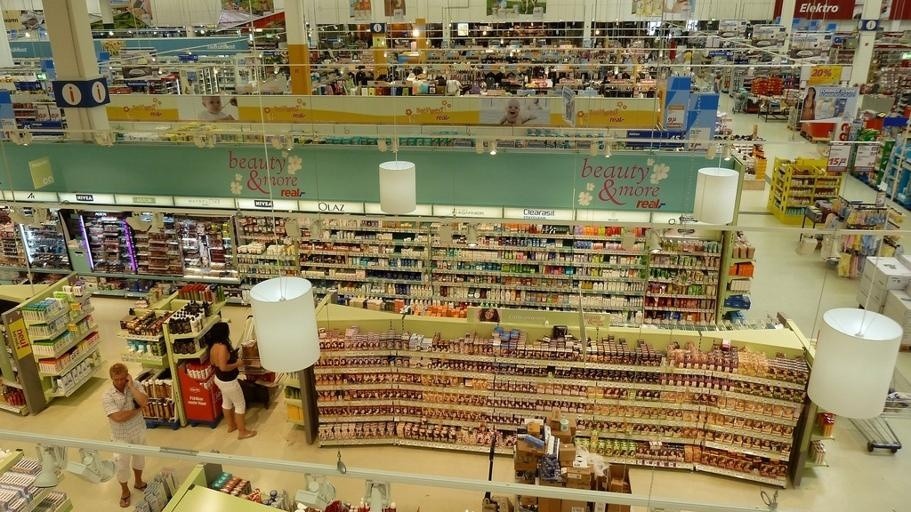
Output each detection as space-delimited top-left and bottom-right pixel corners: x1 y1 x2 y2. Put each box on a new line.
133 483 147 491
120 490 131 508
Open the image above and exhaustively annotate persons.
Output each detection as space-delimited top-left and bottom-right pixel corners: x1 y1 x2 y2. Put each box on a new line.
479 309 501 322
842 124 853 133
198 96 236 121
840 132 850 141
209 321 258 440
186 79 196 94
498 99 537 126
102 363 150 507
802 87 817 120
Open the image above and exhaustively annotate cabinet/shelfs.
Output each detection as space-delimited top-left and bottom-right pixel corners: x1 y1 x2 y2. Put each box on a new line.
767 155 905 279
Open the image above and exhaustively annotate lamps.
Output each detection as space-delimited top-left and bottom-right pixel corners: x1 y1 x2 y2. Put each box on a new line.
693 2 740 225
249 2 322 375
379 2 416 214
806 138 905 420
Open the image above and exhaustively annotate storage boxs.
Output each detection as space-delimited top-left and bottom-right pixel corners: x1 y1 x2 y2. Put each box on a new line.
856 254 911 352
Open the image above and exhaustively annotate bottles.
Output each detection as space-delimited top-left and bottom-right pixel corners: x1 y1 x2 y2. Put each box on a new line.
263 489 298 512
118 283 229 423
0 207 242 300
313 325 811 481
340 495 397 512
121 128 645 149
771 164 842 215
809 412 837 464
240 216 776 333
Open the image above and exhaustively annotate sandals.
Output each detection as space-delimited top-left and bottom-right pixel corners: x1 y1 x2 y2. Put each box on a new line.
238 431 258 440
226 424 237 433
